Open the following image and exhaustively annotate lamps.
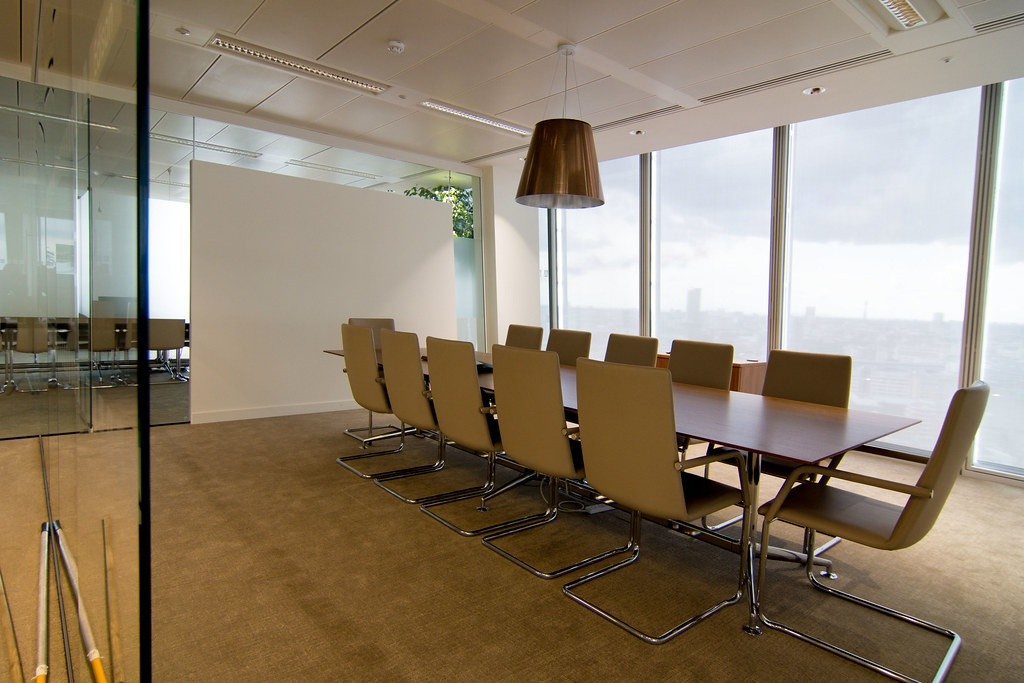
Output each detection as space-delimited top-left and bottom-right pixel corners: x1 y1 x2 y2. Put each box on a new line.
515 43 604 209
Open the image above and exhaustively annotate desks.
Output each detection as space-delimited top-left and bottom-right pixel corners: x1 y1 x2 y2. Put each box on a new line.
324 344 922 638
1 316 90 379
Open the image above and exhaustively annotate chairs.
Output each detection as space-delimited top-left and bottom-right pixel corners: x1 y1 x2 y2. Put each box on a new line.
334 316 852 645
0 300 190 394
757 380 990 683
